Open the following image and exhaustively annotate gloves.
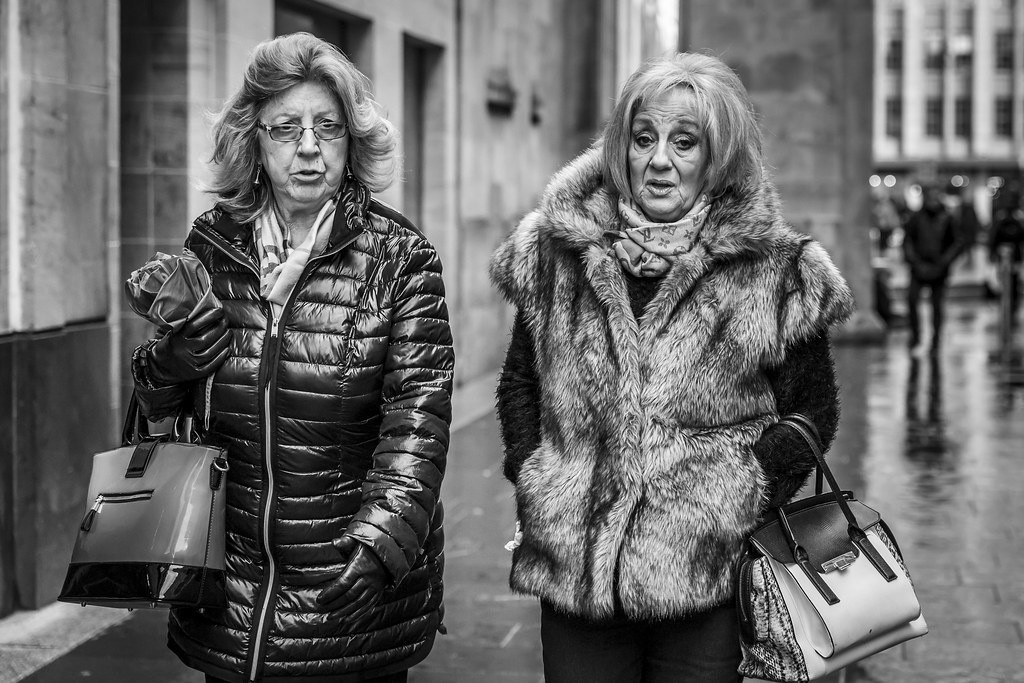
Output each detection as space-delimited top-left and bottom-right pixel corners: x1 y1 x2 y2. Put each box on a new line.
316 535 389 621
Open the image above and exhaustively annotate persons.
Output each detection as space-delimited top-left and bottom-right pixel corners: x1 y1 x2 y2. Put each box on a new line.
898 175 1024 352
129 34 453 683
489 51 856 683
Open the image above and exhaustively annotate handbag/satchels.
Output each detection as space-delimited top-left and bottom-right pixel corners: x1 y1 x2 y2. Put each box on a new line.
148 308 234 386
737 415 930 683
55 380 229 613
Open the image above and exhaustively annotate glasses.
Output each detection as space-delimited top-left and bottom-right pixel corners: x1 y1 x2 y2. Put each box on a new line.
257 120 349 142
